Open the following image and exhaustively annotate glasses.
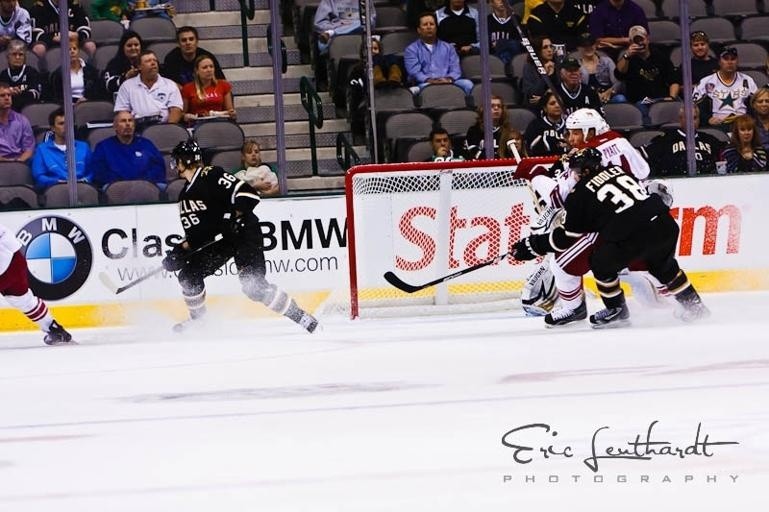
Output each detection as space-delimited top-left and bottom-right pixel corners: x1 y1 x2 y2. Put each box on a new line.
690 32 704 37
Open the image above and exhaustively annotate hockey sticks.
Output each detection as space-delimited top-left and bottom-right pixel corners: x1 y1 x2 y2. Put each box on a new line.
98 232 232 294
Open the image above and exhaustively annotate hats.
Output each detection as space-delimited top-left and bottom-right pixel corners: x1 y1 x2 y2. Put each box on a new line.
719 46 737 57
561 57 581 68
629 26 647 42
576 34 595 47
691 31 709 42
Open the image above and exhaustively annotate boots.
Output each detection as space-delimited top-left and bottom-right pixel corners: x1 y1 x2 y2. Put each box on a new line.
590 290 629 324
545 289 586 324
172 304 205 333
667 284 710 322
44 319 71 344
286 298 317 333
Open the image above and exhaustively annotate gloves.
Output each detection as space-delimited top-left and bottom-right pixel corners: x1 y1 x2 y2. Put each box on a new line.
163 244 193 271
511 235 547 260
222 215 246 240
513 158 547 181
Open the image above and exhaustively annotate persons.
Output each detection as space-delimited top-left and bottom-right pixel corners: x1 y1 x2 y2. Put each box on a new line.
1 2 236 195
229 140 280 198
510 146 713 330
0 225 78 348
543 109 674 327
161 139 324 338
519 146 670 317
346 37 403 146
400 1 768 176
313 1 378 54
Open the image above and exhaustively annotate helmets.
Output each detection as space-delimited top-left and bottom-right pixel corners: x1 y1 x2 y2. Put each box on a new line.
567 147 602 177
563 108 610 137
639 179 673 207
170 139 201 178
